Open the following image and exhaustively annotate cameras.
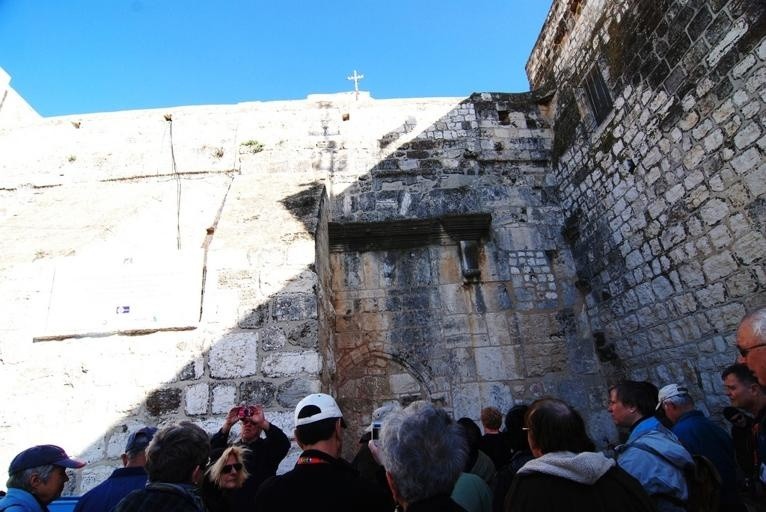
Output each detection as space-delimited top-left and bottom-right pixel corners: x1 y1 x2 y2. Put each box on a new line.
239 409 253 419
372 423 381 445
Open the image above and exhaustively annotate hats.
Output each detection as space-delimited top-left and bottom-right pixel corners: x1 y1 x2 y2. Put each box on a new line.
9 445 85 476
124 426 158 452
655 383 688 411
295 393 347 429
723 407 738 419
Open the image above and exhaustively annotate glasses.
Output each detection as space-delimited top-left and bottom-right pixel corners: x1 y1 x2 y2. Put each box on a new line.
223 463 243 473
736 344 765 358
